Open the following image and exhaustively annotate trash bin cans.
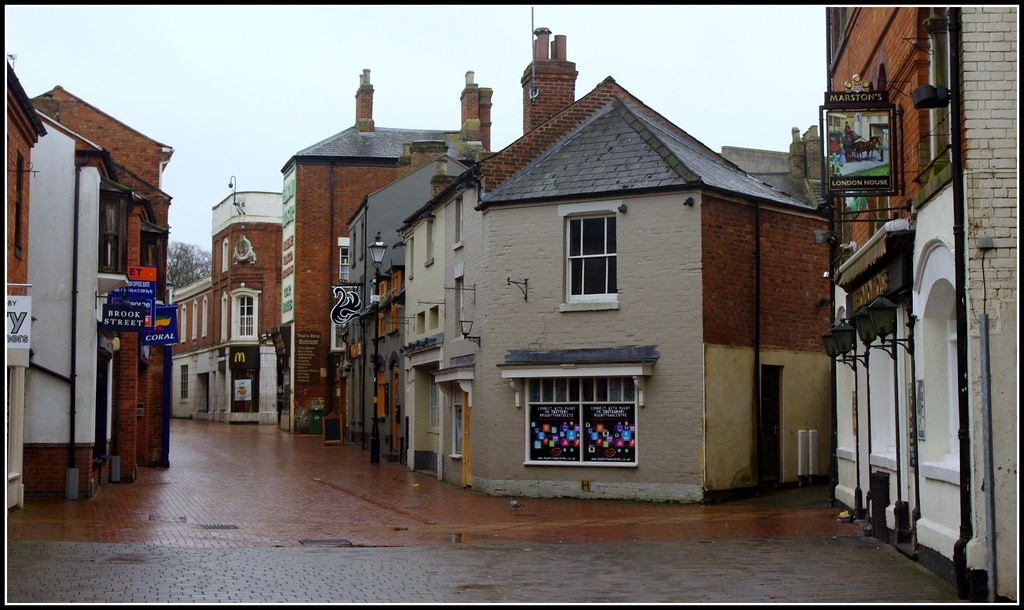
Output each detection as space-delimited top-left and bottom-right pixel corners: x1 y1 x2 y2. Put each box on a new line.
308 406 324 434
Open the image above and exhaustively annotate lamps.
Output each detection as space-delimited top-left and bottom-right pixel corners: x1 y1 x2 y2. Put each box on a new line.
820 324 856 372
852 305 895 360
391 241 406 250
865 292 914 357
810 229 837 249
458 320 481 349
911 84 950 110
418 213 437 220
830 319 868 369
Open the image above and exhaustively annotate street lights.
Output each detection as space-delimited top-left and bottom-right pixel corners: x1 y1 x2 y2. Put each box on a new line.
366 230 389 463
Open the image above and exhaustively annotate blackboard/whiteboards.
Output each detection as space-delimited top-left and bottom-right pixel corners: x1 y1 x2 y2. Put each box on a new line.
322 412 343 443
